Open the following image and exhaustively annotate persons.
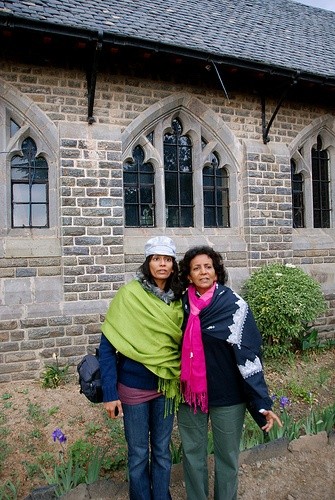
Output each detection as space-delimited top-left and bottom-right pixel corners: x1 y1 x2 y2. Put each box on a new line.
98 236 184 500
177 246 284 500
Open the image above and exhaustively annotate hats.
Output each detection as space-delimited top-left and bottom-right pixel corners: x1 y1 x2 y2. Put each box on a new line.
145 236 177 258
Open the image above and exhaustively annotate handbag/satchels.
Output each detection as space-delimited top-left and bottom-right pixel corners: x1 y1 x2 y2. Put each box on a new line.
77 347 103 403
